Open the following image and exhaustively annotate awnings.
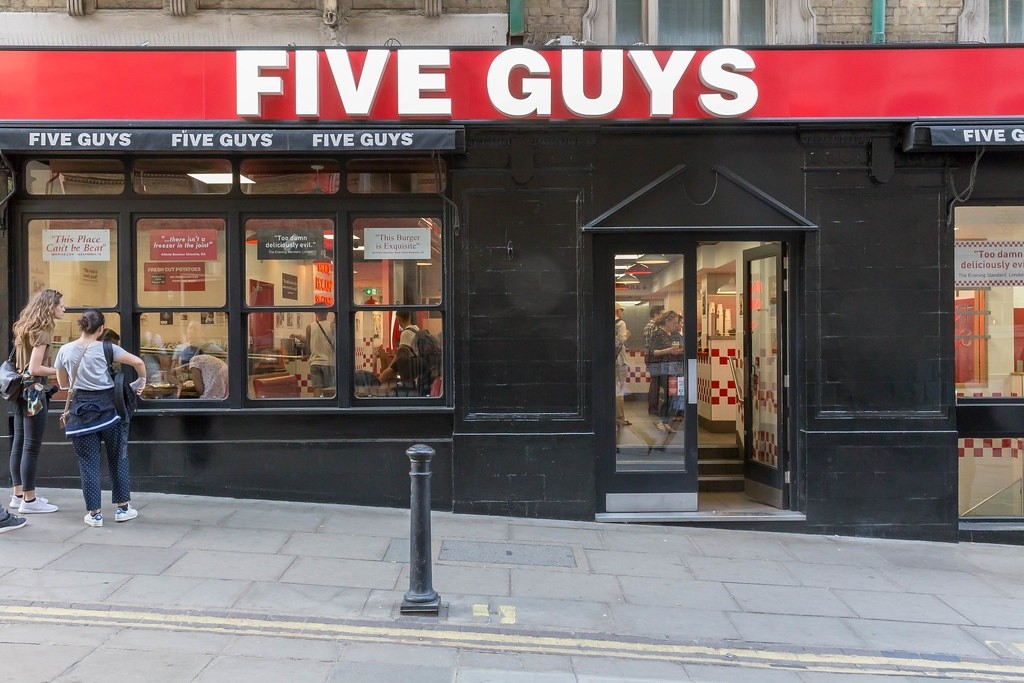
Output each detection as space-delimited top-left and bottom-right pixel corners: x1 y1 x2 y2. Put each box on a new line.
0 126 466 192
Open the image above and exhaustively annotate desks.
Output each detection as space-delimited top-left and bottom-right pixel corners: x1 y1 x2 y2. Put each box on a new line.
142 382 199 399
321 382 396 397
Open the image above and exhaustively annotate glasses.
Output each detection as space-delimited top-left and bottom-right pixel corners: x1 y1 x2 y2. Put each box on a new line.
618 307 624 313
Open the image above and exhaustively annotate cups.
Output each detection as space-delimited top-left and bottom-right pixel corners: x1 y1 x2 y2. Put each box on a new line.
708 302 732 336
672 342 679 349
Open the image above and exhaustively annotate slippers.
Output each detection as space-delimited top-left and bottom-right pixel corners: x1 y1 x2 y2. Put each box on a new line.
651 419 677 434
622 420 633 426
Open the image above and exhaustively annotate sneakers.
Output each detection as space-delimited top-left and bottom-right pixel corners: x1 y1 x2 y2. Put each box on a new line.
18 499 59 514
0 513 27 533
9 495 49 508
115 501 139 522
84 509 104 527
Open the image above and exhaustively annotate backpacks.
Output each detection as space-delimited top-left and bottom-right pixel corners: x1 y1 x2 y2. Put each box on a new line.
405 327 442 373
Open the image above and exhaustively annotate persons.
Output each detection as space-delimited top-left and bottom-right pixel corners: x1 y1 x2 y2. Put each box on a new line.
432 366 443 397
0 503 26 533
379 310 431 397
643 306 684 421
180 346 228 399
140 313 228 384
10 289 65 513
615 303 631 425
289 302 336 397
53 309 147 526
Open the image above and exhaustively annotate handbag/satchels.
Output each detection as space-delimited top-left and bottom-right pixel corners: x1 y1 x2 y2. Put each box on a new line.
59 410 70 430
102 339 138 420
0 360 23 402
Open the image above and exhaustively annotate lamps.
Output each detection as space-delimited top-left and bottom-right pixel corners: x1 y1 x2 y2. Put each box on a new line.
716 271 736 293
615 282 629 289
615 274 639 283
623 264 652 274
635 253 669 263
306 165 328 193
363 294 377 304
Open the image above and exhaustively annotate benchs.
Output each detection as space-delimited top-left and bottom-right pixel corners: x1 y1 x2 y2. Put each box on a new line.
248 371 302 400
